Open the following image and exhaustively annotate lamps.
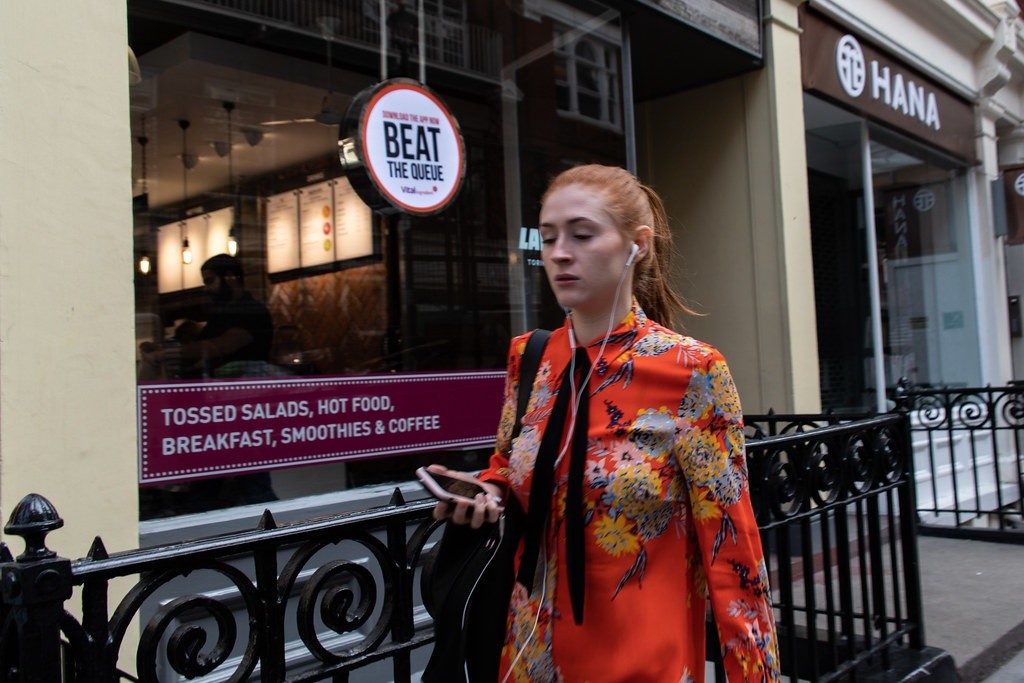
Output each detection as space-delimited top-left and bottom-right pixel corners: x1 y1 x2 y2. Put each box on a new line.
245 128 264 147
137 137 150 273
213 142 233 158
222 103 238 257
182 155 199 170
178 118 192 264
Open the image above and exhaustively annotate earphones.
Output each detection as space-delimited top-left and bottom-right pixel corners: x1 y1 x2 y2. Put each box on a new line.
626 244 639 266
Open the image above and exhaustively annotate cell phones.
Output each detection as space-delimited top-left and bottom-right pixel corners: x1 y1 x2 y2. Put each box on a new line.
417 467 505 511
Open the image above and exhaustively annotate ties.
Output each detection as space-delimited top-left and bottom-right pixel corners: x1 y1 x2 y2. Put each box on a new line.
515 349 591 626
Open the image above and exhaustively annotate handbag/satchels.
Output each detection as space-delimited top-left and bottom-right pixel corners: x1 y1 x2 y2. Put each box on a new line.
421 488 522 645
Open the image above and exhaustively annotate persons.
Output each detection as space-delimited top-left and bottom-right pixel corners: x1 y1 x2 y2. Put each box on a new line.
137 254 273 382
427 164 780 683
